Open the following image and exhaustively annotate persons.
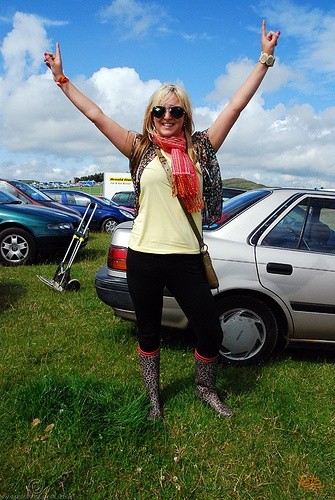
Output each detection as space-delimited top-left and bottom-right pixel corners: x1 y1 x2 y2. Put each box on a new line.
43 19 281 421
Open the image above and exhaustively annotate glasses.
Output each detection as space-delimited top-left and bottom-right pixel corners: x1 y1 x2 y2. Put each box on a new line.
150 106 186 119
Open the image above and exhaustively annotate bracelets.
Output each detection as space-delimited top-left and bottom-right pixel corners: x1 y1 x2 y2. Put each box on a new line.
259 51 275 67
55 73 70 89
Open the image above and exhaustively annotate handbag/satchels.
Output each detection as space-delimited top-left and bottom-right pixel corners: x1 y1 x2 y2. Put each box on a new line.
201 247 220 290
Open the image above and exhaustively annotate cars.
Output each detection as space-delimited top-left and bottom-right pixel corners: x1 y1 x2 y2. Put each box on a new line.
93 186 335 369
0 189 90 266
0 179 83 219
201 187 335 253
109 190 137 217
31 189 135 235
58 194 136 217
28 179 103 189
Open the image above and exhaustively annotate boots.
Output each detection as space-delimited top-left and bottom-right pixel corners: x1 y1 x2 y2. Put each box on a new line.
137 346 163 424
194 350 234 417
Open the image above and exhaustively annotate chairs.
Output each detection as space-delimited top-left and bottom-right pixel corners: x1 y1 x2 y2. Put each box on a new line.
294 221 335 253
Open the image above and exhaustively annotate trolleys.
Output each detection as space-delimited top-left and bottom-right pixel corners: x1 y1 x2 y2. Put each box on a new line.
35 201 98 294
